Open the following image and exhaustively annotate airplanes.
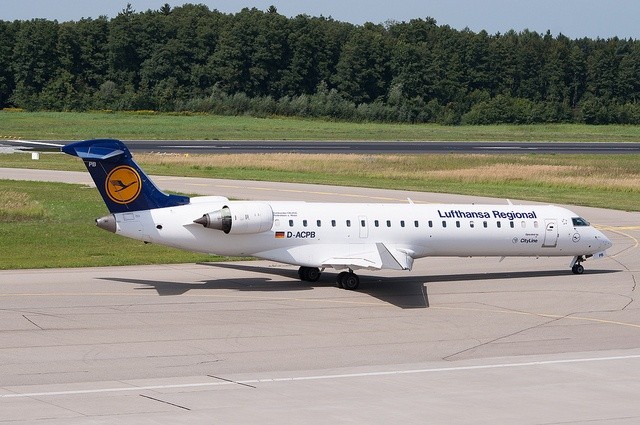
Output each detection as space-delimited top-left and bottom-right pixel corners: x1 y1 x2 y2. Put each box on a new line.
60 138 613 290
